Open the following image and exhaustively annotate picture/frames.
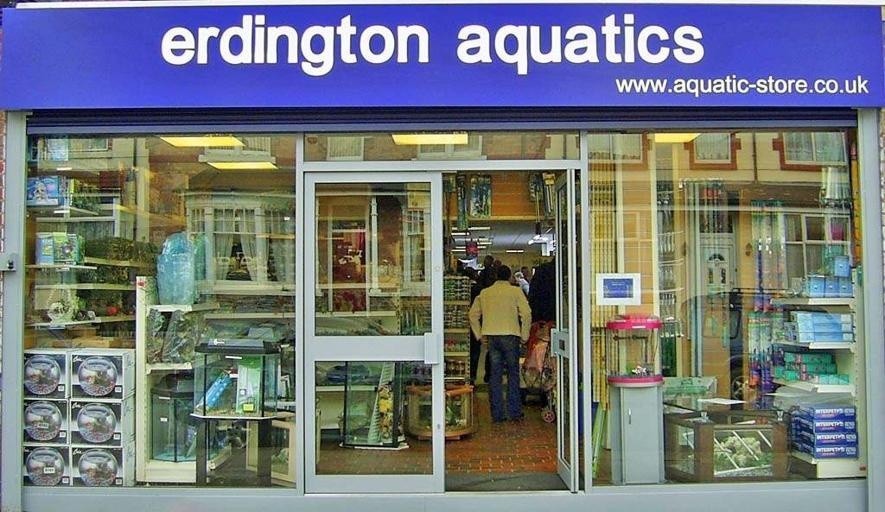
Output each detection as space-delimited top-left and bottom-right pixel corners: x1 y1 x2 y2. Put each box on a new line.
43 138 113 159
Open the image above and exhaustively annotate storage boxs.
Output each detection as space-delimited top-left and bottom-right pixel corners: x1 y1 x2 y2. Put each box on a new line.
22 347 137 487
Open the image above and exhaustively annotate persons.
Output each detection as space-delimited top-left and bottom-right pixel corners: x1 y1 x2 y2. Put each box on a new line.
466 252 555 423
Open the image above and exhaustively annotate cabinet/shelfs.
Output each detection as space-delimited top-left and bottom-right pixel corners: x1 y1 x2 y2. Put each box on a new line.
400 276 472 383
135 277 232 483
608 381 668 486
196 280 397 431
26 204 187 328
771 268 868 480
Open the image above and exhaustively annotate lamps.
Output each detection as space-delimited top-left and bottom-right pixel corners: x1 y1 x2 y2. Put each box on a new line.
155 128 248 149
198 135 279 173
389 130 470 146
